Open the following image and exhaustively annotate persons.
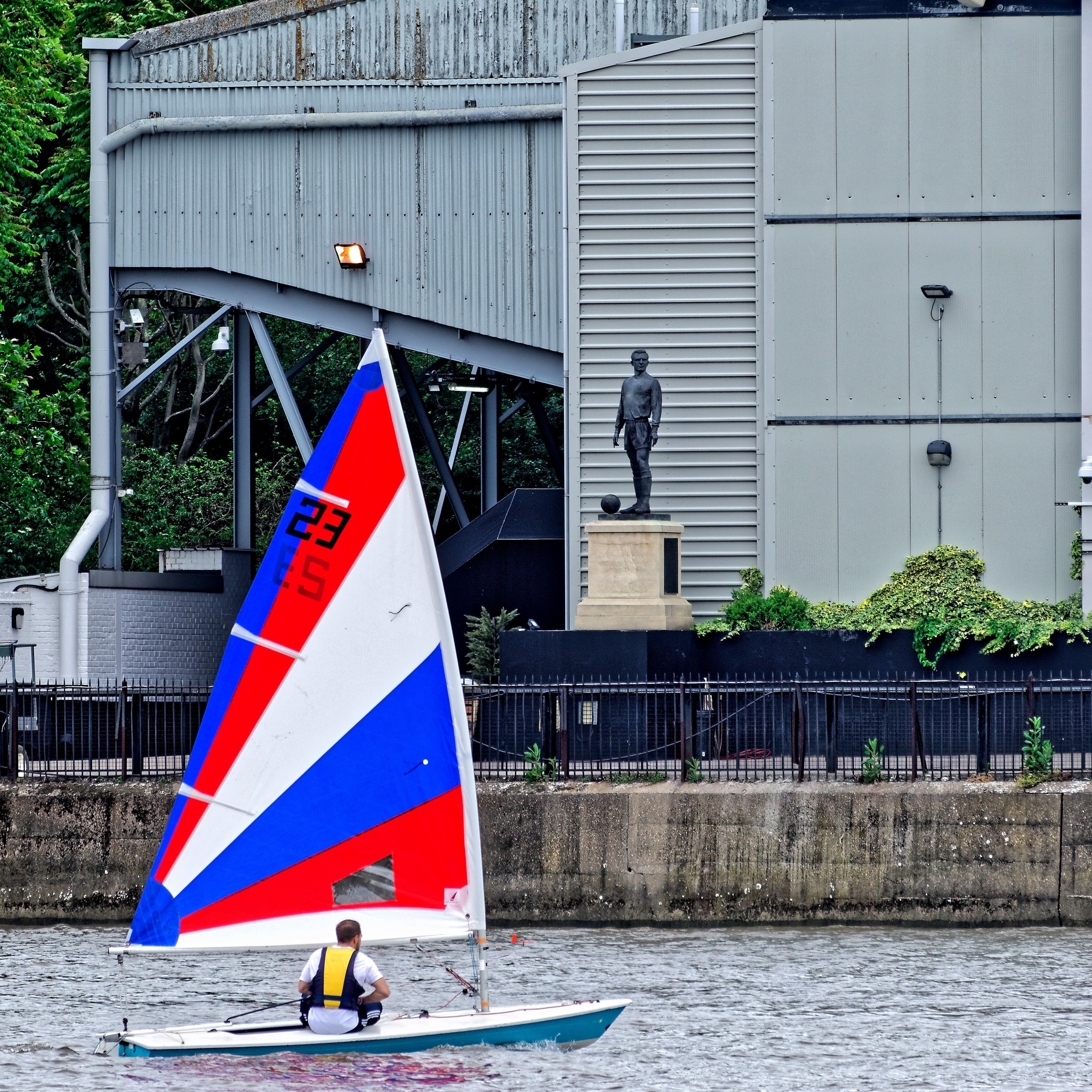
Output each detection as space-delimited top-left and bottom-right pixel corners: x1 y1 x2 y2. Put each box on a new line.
297 920 391 1035
613 350 662 515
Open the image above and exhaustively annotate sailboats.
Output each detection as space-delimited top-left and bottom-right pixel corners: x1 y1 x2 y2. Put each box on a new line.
93 326 631 1060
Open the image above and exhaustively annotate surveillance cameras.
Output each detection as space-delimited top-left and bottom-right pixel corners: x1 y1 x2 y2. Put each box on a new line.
129 309 144 328
212 339 230 357
1078 466 1092 485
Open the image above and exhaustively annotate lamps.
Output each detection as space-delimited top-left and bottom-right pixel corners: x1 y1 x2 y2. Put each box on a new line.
920 284 953 299
211 316 230 353
333 243 368 268
429 368 440 392
927 440 951 466
527 619 539 630
445 377 490 395
11 608 25 630
